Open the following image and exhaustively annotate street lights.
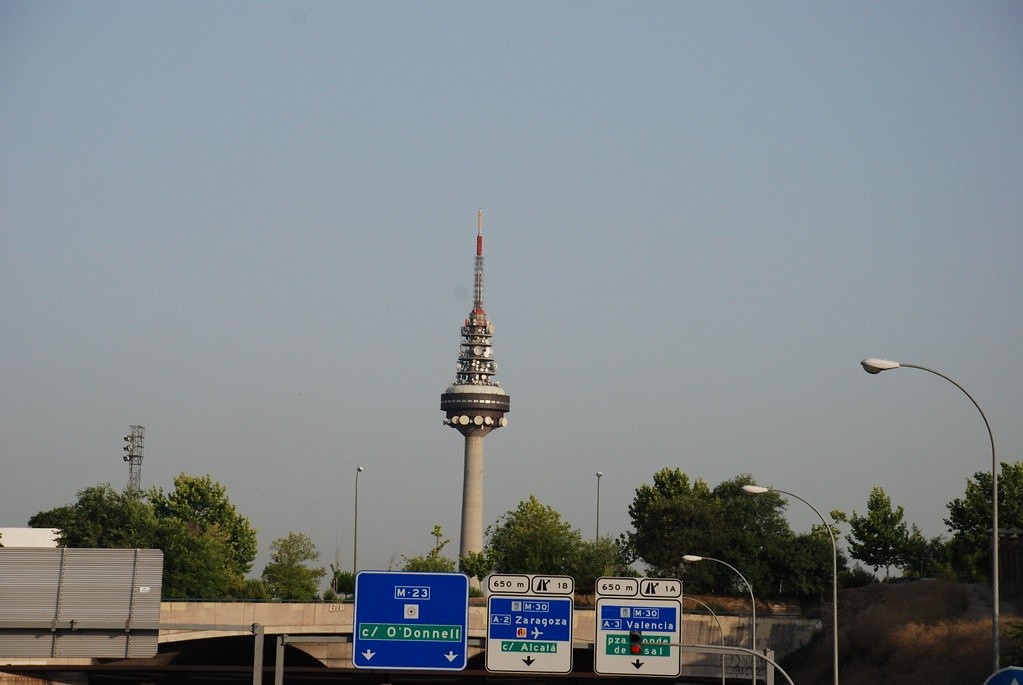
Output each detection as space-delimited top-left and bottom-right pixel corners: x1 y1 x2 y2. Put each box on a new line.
594 471 603 542
352 464 363 575
858 354 1006 671
682 553 758 684
741 483 841 685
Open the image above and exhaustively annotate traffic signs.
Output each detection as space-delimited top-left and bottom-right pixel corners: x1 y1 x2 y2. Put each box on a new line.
485 595 573 674
595 598 681 676
353 569 467 671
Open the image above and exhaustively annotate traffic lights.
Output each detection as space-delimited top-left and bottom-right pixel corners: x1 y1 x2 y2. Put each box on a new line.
629 630 642 655
